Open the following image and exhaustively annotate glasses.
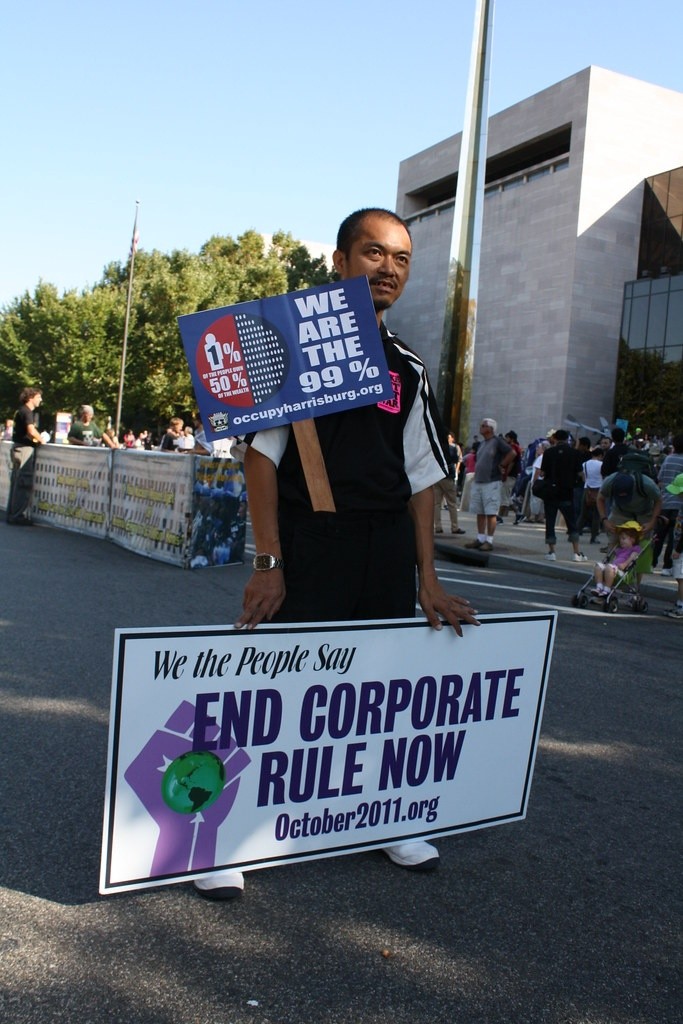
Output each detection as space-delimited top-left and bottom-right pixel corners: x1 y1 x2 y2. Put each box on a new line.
482 424 491 428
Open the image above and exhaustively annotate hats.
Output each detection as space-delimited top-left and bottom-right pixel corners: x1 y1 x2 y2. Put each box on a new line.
665 474 683 494
507 430 517 440
615 521 645 540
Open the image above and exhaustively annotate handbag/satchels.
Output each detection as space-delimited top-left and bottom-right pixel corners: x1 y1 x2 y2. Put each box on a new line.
586 488 598 507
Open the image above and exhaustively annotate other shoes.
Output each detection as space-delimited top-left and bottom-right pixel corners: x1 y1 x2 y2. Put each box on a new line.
664 604 679 615
599 591 610 597
452 528 466 534
591 588 602 596
600 546 610 553
496 516 547 527
10 518 33 526
589 538 601 545
436 529 443 533
661 568 672 575
669 606 683 618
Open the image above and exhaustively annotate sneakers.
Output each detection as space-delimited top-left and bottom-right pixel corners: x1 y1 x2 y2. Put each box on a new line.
573 553 587 562
465 539 483 548
194 871 244 899
384 840 440 872
545 552 556 560
479 541 493 551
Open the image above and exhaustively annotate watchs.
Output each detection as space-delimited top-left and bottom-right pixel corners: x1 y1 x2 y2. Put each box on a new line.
252 552 284 571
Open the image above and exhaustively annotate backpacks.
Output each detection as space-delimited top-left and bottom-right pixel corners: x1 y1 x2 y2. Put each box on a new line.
612 453 659 497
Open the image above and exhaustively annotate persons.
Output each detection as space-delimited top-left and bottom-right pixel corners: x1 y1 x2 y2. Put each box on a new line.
539 430 593 562
67 405 119 449
464 419 517 550
6 387 47 525
433 427 683 576
664 473 683 617
591 528 642 598
192 207 482 900
0 412 247 454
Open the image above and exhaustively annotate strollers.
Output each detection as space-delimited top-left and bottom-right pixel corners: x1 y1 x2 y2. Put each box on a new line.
571 511 669 615
